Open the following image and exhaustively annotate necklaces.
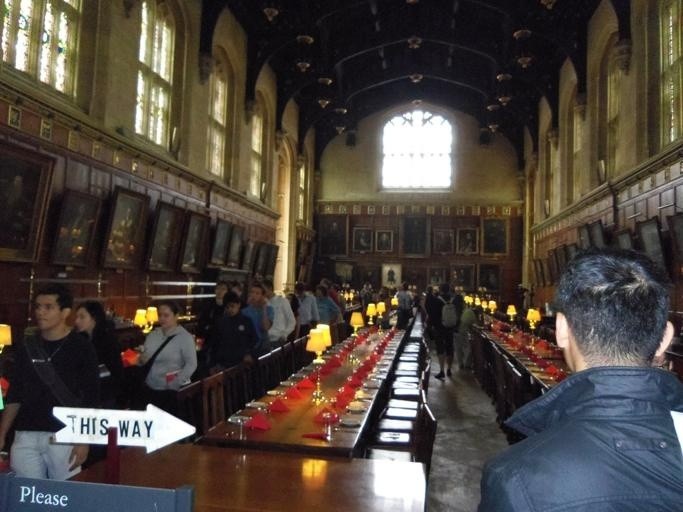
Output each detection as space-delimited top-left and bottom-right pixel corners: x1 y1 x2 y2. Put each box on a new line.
40 330 70 362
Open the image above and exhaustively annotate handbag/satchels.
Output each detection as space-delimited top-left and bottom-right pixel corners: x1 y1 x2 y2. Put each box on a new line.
133 361 153 379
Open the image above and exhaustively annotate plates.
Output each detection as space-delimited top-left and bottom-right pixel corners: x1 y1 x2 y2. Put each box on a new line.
227 326 369 425
508 346 558 387
338 328 406 427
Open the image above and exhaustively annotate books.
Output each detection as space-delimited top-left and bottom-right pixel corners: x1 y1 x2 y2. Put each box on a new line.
165 369 192 388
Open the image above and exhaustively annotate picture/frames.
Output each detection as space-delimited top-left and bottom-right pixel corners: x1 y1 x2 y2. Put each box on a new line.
314 211 507 296
529 212 681 286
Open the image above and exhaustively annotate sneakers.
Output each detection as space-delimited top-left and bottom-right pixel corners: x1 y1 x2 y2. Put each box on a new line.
447 369 451 376
435 371 444 378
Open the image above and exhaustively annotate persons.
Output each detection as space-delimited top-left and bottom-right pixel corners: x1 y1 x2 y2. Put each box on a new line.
118 298 199 412
477 246 683 511
201 269 481 383
0 281 95 481
70 299 123 470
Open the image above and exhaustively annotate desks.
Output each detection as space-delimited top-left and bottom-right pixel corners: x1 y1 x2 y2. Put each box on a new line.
65 442 428 512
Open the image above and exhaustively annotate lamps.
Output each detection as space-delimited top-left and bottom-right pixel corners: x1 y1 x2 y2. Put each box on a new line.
0 323 13 354
133 306 160 335
263 5 552 136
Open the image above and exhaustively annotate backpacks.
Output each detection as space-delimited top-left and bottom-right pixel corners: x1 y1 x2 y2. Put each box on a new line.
438 296 458 327
